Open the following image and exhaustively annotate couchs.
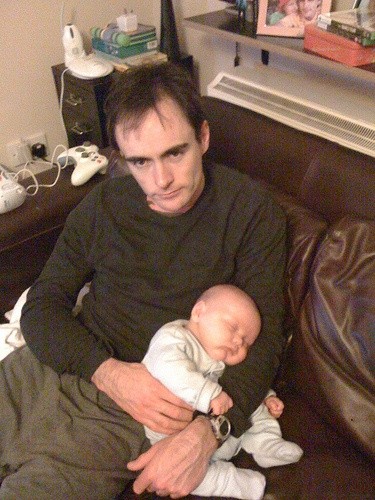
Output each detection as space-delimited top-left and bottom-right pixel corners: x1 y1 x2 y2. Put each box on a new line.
0 91 375 500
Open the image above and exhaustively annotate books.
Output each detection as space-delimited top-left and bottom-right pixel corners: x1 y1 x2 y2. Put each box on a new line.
317 8 375 46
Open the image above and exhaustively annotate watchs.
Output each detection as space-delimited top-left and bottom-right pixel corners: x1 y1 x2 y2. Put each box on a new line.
195 414 231 448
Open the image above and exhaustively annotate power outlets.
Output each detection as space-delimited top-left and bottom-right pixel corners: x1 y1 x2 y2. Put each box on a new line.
8 132 50 167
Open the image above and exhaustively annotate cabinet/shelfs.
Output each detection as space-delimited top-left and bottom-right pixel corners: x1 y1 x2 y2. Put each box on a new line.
51 47 194 151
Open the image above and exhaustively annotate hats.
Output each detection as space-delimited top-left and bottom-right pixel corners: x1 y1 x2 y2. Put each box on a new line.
278 0 290 12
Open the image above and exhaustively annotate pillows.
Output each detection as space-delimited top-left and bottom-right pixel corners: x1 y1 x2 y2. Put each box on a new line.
284 213 375 464
246 173 329 387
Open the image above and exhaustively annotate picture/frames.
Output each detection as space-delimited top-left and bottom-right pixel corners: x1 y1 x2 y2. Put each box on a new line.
256 0 332 37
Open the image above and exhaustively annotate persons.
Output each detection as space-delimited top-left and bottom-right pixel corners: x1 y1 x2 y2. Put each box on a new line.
142 285 304 500
292 0 320 27
269 0 298 25
0 59 286 500
